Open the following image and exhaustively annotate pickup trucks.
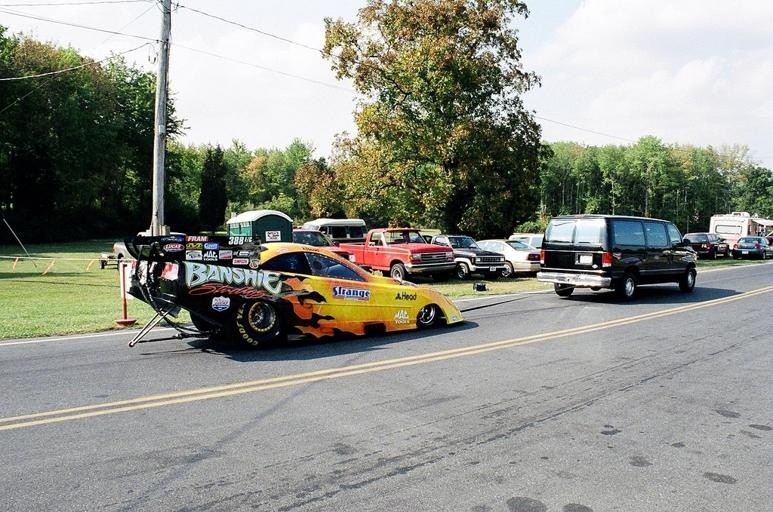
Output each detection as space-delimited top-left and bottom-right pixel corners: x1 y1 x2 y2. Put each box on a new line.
394 235 505 281
338 227 456 281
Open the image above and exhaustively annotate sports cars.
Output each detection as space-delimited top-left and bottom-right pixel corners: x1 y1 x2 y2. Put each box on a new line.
124 233 464 353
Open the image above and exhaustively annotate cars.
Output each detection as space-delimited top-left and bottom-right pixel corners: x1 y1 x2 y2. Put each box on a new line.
731 236 772 260
291 228 349 262
469 239 540 278
681 232 730 260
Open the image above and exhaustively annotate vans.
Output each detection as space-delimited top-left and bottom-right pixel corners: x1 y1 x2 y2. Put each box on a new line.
507 233 543 251
300 218 368 243
536 213 696 302
